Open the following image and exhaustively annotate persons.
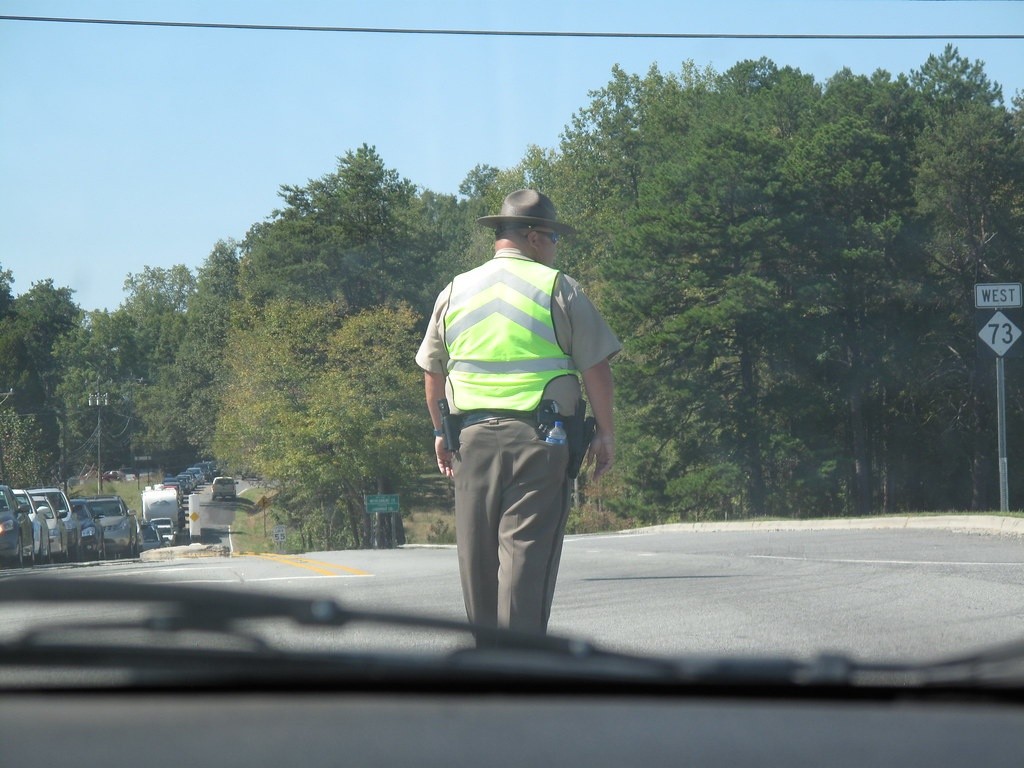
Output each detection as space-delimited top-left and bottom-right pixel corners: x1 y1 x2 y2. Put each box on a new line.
414 188 621 647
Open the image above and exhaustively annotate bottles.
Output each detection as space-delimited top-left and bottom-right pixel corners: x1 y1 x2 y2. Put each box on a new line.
546 421 567 446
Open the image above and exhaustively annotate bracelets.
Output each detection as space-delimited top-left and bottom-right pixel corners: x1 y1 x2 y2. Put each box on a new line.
434 428 442 436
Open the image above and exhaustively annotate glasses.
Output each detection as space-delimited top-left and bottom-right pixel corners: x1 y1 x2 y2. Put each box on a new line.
524 230 555 244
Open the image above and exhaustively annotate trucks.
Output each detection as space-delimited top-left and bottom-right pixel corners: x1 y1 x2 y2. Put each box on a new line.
142 484 179 535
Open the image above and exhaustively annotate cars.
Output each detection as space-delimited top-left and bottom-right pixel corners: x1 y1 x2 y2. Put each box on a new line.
212 476 239 500
164 472 198 502
194 463 212 482
69 499 106 560
17 496 70 563
0 489 52 565
27 488 84 562
81 494 138 559
186 468 205 484
157 525 177 546
142 524 169 550
1 485 35 569
203 461 221 477
101 467 136 481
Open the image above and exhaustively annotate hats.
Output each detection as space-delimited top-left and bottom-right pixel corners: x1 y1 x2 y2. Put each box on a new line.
476 189 574 234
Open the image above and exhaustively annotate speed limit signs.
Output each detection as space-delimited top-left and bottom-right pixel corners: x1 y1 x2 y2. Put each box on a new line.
273 525 286 542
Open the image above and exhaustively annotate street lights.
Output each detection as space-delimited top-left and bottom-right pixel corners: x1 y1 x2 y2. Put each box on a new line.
88 392 109 494
